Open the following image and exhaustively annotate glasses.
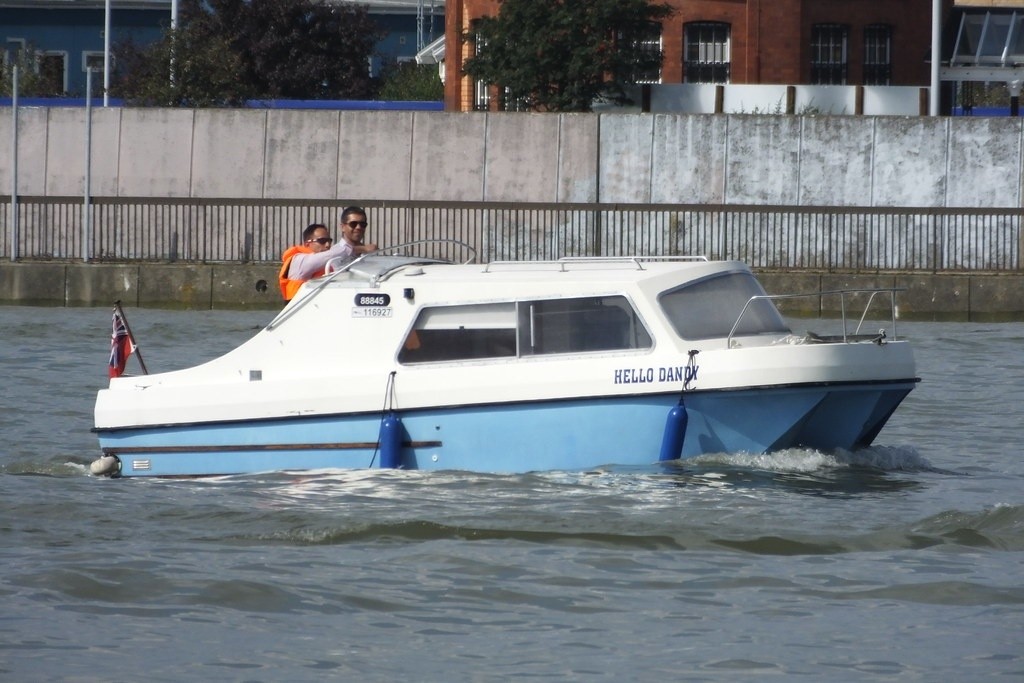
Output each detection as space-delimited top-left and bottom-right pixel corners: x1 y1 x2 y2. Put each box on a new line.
345 221 369 229
305 238 333 245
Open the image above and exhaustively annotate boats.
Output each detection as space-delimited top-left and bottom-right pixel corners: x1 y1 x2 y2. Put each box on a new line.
91 236 923 478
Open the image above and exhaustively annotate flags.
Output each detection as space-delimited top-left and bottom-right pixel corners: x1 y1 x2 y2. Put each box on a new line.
108 306 138 380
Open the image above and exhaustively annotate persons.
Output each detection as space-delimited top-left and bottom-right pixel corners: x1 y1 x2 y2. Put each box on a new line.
279 224 380 306
328 205 369 273
